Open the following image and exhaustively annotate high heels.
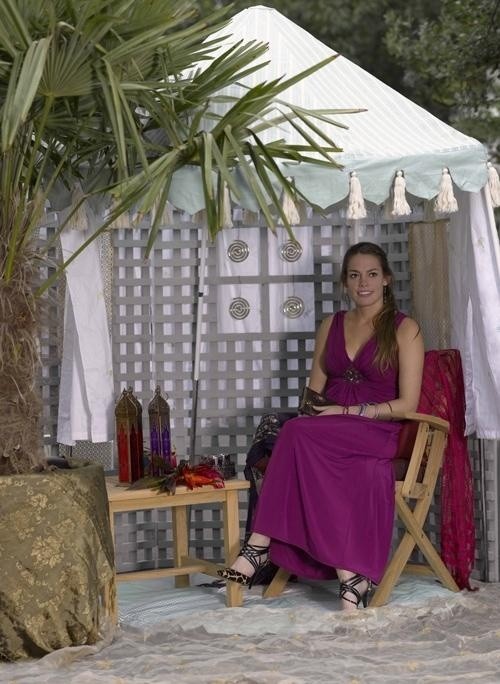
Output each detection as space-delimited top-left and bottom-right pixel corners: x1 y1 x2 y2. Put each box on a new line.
217 543 272 589
339 573 375 607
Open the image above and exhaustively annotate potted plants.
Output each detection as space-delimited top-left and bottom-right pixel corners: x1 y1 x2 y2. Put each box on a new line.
0 0 367 663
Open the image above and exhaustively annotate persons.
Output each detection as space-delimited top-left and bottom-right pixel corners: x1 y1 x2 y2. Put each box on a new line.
216 239 427 614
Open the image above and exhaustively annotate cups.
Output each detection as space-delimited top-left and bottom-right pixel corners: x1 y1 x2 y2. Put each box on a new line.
200 452 230 465
208 462 235 479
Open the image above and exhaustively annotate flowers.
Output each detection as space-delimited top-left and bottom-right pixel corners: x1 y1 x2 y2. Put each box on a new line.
124 454 225 496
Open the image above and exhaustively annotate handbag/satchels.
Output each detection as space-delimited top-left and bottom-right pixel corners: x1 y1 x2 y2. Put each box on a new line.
246 413 300 478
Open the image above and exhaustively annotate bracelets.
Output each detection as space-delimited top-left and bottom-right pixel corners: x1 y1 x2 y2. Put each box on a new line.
357 401 380 419
345 404 349 415
342 407 345 414
385 401 394 422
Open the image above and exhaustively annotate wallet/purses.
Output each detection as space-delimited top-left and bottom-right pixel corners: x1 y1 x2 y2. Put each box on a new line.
298 387 338 416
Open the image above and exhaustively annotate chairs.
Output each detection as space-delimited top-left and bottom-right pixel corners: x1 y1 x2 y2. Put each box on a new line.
255 349 460 606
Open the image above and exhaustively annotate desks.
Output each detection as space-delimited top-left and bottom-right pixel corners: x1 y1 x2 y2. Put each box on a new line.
107 479 252 606
